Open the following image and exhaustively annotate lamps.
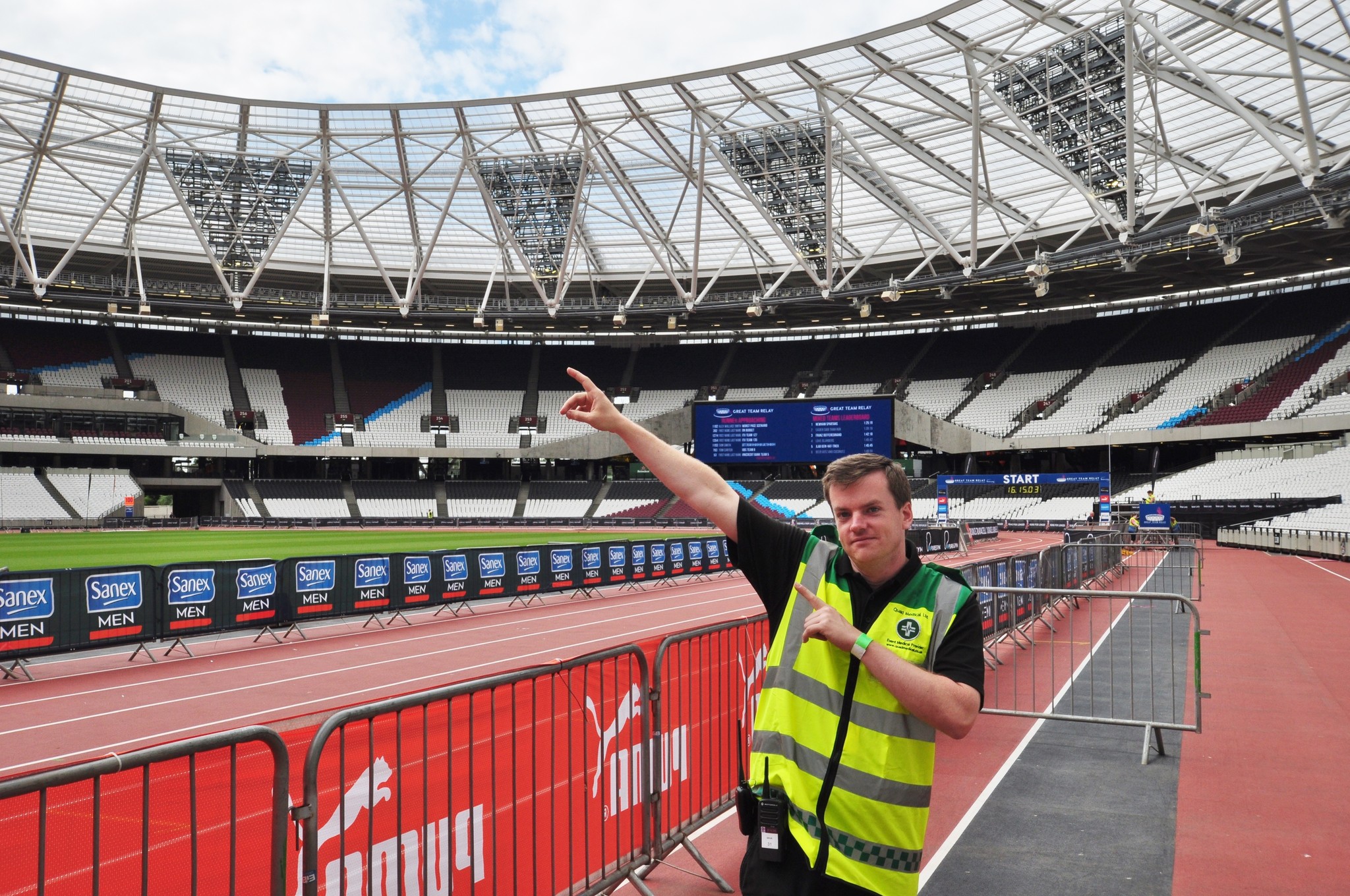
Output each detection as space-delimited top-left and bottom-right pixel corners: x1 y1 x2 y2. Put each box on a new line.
1025 244 1050 297
1187 215 1241 266
881 273 901 302
613 300 626 326
746 299 763 317
139 293 150 315
311 311 330 326
473 302 485 327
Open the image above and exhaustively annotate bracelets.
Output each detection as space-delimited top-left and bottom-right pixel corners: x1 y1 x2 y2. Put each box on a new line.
851 633 873 660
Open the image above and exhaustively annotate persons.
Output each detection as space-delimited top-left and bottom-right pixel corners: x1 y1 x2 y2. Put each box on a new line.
560 367 986 896
1142 490 1155 504
1128 513 1139 550
1229 400 1235 406
170 512 176 518
428 509 432 526
240 422 246 430
1170 517 1181 549
1087 511 1094 526
1341 388 1348 395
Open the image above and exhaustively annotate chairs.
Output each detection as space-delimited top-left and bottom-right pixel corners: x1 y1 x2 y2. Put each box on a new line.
0 333 1350 538
